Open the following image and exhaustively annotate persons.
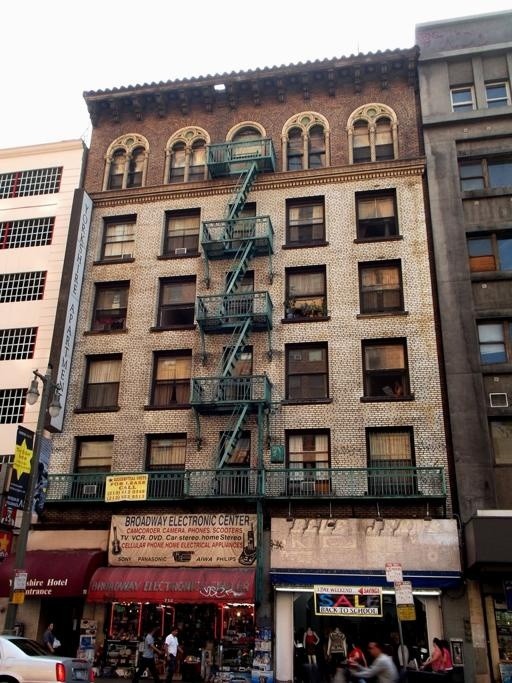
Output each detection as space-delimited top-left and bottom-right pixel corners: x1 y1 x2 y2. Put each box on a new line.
0 506 14 526
301 627 322 666
343 630 455 683
325 627 349 661
160 626 185 682
131 625 162 682
42 622 57 656
198 628 218 682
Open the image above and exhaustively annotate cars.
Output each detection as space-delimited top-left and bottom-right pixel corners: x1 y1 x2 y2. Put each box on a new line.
1 636 93 683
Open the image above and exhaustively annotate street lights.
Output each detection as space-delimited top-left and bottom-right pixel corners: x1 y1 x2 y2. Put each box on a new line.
2 362 64 634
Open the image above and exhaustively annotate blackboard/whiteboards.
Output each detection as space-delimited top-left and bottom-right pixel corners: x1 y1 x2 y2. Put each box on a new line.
105 602 142 666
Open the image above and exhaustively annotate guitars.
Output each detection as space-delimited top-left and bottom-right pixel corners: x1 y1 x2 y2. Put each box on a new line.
111 527 122 555
